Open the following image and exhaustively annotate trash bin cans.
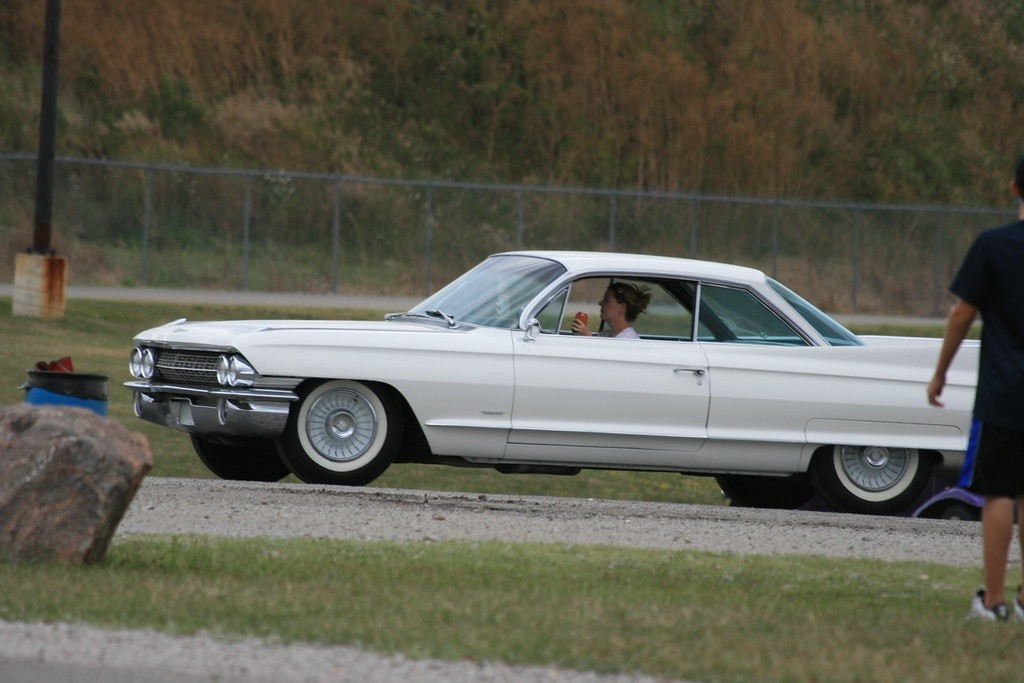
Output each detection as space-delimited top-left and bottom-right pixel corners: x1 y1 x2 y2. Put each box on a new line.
22 369 110 415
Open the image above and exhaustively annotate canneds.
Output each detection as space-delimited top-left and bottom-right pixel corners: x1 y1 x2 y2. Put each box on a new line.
572 312 588 332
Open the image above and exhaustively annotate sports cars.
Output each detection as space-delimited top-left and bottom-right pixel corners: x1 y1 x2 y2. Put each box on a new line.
122 250 982 517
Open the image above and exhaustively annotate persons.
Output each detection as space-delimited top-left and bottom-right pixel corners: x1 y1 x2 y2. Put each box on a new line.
571 280 653 339
928 154 1024 621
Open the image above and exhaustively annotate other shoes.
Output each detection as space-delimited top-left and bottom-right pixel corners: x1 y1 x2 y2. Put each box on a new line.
1013 597 1024 621
974 587 1009 620
